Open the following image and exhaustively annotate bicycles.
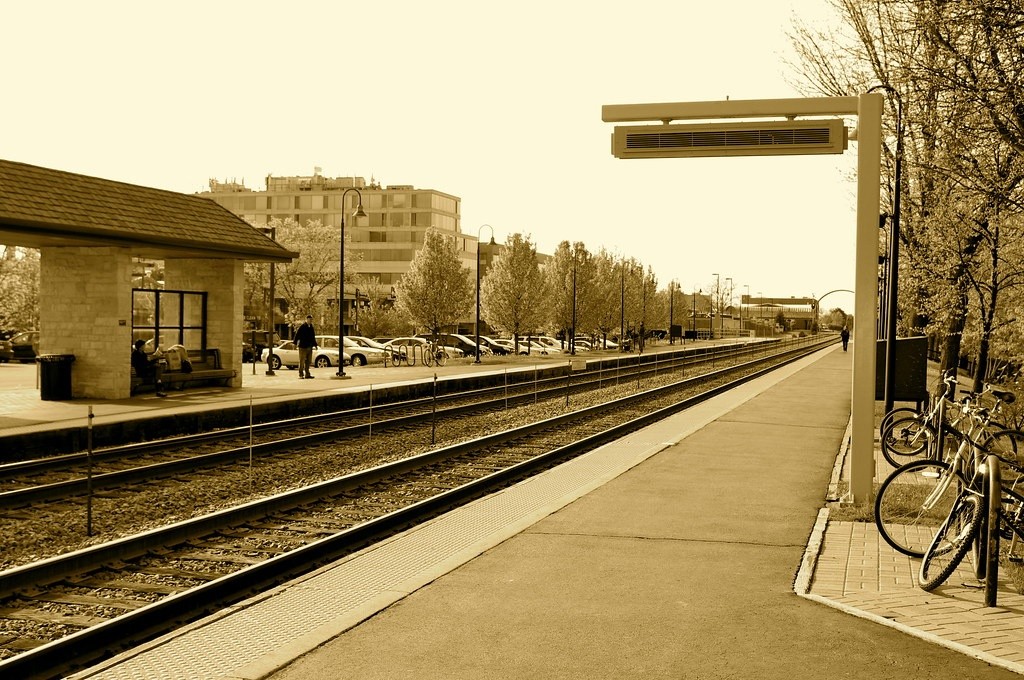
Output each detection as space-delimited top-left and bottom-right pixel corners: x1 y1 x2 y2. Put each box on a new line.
874 368 1024 591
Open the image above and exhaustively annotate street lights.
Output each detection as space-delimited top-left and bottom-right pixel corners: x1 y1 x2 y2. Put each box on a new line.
712 273 773 338
670 277 681 344
693 283 702 341
331 188 367 379
570 250 589 355
848 84 902 439
256 227 276 375
620 261 635 340
471 224 499 365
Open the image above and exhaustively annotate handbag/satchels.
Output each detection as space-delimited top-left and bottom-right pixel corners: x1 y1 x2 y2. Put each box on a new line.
164 344 195 374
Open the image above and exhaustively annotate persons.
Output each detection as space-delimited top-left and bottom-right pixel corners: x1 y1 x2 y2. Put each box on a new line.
131 339 167 398
357 340 362 347
638 324 645 347
293 315 318 379
840 325 850 352
246 339 258 363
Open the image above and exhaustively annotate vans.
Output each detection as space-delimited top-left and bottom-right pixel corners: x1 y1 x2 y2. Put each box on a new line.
511 336 596 352
413 333 493 358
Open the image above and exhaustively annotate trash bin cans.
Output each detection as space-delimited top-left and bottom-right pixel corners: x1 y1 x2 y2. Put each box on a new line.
36 354 76 401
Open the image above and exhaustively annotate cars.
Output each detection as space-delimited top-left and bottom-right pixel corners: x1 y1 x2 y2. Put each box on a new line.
0 331 40 363
465 334 561 356
261 335 464 370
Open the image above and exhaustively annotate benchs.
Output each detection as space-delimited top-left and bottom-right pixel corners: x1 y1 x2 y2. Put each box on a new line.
131 347 238 396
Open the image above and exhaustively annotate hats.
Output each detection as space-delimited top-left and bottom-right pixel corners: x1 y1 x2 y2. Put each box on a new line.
135 340 146 349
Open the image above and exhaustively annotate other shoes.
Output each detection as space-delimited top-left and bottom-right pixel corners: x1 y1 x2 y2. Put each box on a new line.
299 375 304 379
156 391 167 397
306 375 315 378
